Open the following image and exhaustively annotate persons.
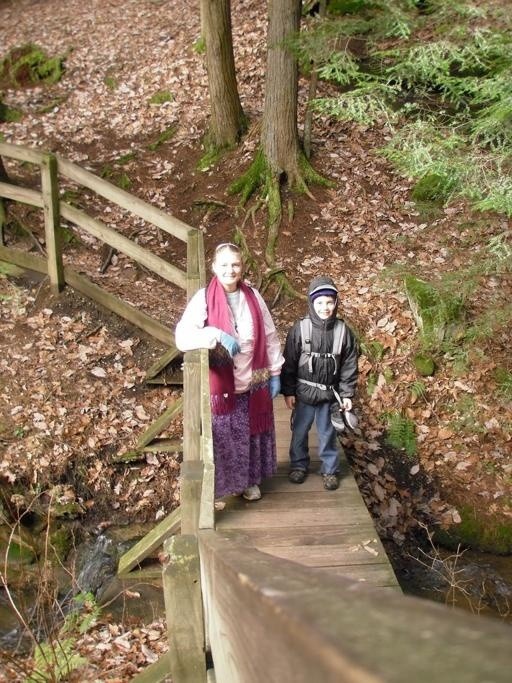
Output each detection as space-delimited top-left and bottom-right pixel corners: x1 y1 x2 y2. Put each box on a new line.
279 275 360 492
174 242 285 508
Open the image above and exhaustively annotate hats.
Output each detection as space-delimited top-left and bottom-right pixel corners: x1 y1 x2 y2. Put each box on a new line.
311 289 338 302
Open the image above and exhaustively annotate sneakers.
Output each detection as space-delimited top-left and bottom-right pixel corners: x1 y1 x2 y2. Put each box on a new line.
289 469 305 483
242 483 262 501
321 473 339 490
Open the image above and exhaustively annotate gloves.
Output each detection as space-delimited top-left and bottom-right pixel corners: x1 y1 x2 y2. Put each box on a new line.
269 375 281 399
219 332 241 358
